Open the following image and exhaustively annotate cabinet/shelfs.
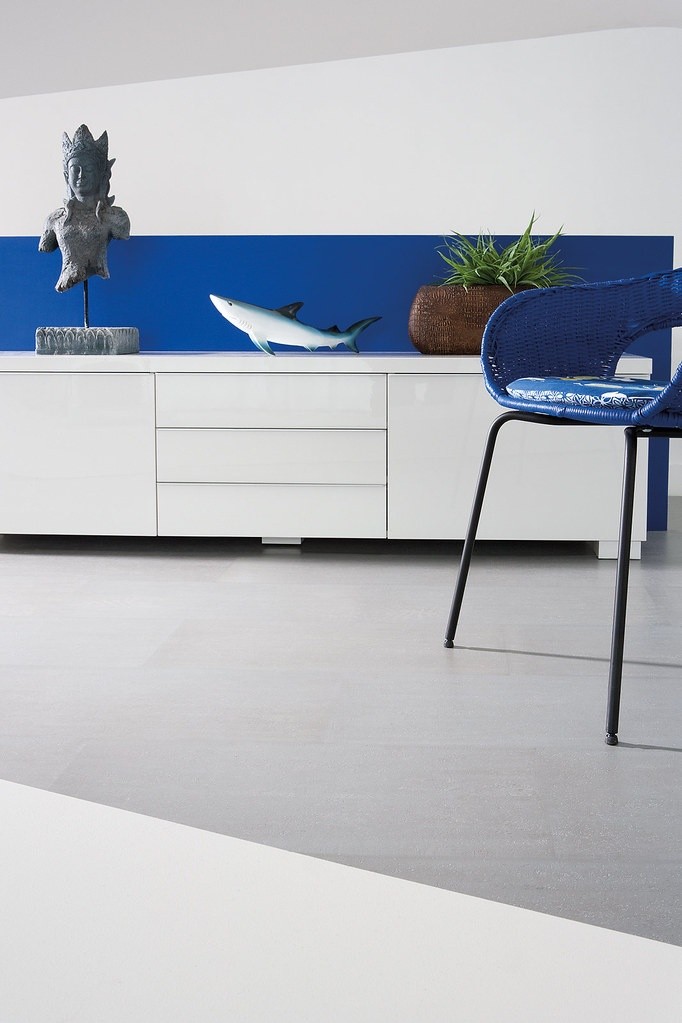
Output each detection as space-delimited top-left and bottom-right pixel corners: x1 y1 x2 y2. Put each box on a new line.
0 348 655 560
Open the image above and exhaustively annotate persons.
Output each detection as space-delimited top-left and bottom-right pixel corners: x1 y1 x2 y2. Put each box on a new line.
38 124 130 294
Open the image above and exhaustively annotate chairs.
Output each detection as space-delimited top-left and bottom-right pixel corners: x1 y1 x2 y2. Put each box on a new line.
443 268 682 745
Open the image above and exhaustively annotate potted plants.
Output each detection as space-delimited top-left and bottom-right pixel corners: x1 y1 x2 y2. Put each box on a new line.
408 209 591 355
